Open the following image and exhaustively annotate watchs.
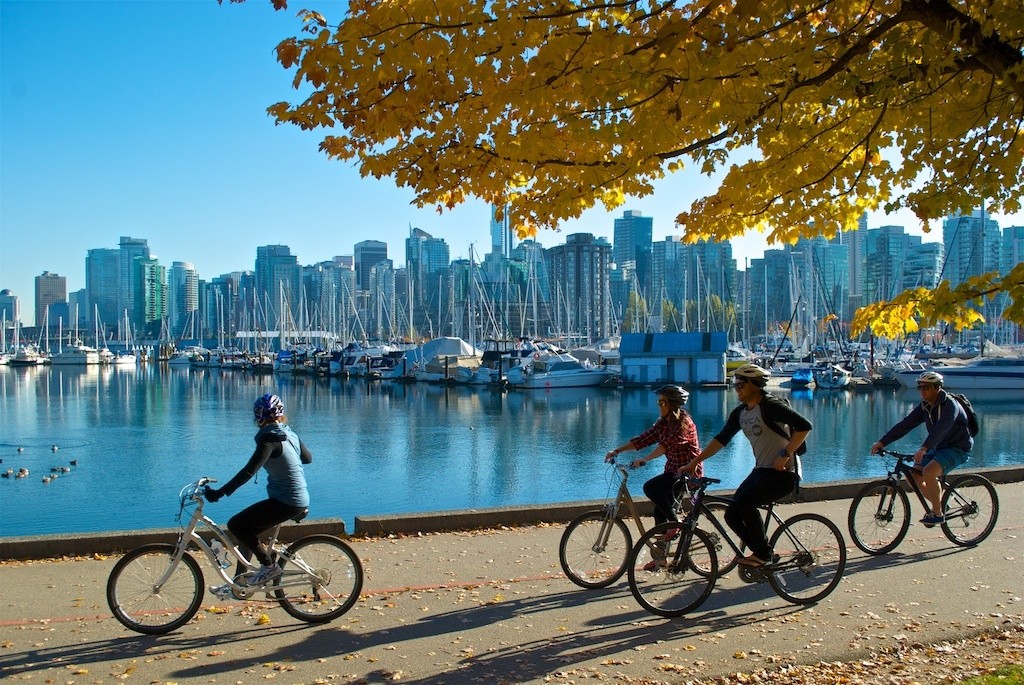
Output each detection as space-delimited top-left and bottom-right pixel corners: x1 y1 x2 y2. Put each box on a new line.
780 449 790 457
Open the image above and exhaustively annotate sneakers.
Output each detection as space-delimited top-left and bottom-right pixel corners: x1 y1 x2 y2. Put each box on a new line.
209 585 234 598
249 562 283 584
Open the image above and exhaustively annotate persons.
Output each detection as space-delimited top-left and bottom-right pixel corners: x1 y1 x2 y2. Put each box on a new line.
605 384 703 570
677 365 813 568
205 394 312 599
871 372 974 525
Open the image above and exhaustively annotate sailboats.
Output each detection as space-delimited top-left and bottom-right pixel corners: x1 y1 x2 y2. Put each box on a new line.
726 210 1021 389
0 259 620 389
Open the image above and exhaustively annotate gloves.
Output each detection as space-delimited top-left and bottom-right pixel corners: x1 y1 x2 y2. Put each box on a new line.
204 483 224 503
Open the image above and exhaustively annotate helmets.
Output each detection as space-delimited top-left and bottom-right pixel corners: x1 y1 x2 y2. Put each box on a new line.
655 383 690 405
253 393 283 419
914 371 944 385
729 364 771 380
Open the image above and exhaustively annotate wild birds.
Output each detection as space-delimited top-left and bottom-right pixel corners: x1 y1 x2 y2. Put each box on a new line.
0 445 77 483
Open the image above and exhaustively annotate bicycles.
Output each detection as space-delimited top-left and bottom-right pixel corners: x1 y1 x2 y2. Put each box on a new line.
558 456 747 590
105 478 363 635
627 470 847 617
848 448 1000 556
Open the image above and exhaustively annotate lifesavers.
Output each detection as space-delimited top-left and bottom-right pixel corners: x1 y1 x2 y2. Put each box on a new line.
869 370 873 376
534 353 539 360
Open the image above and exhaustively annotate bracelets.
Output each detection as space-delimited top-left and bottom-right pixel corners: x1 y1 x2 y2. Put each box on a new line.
614 450 620 454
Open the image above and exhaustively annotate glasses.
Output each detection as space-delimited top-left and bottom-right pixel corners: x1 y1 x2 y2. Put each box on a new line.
917 385 929 390
731 383 744 389
655 399 667 407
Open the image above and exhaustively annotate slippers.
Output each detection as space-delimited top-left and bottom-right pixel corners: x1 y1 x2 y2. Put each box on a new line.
736 556 773 567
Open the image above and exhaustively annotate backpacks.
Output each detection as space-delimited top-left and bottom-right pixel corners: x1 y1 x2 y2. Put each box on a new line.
951 392 979 437
760 393 807 456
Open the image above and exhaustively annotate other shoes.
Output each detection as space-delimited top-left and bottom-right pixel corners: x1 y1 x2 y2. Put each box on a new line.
918 513 945 524
664 519 683 542
643 561 667 570
